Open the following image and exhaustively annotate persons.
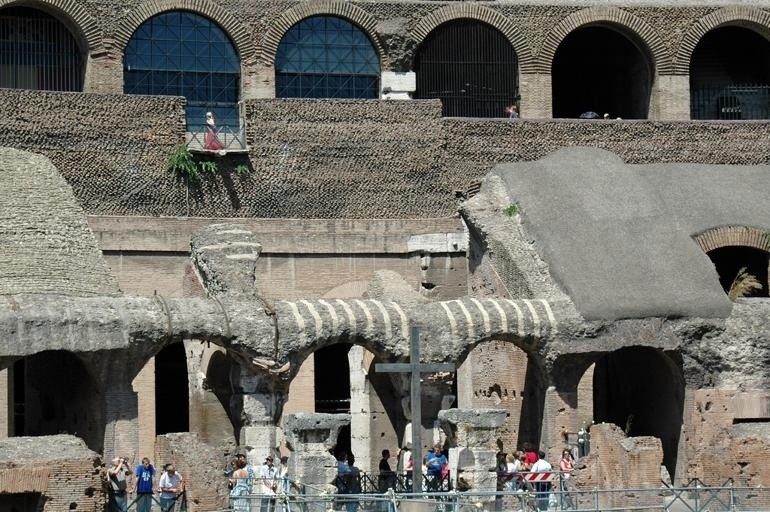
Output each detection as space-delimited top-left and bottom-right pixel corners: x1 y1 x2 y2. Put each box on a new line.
509 106 518 119
333 452 365 512
495 442 577 512
157 464 171 504
109 457 133 512
158 464 185 511
378 444 452 511
205 112 225 150
131 457 158 512
224 453 295 512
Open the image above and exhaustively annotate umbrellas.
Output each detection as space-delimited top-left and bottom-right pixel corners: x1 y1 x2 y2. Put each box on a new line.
579 111 599 118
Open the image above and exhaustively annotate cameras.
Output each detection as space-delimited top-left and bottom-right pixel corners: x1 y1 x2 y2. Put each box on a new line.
123 458 129 463
